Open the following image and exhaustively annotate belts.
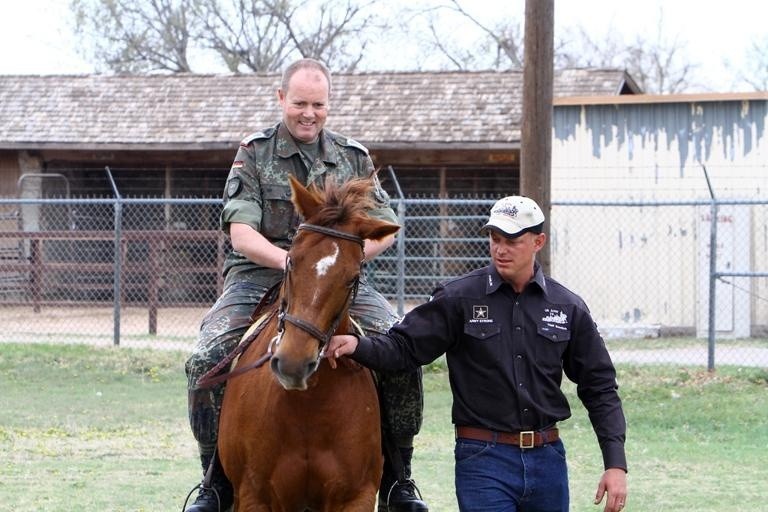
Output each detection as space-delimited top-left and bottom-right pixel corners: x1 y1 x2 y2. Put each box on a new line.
457 425 559 449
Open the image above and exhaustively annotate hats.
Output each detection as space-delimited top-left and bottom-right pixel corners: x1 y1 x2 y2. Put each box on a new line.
476 196 545 240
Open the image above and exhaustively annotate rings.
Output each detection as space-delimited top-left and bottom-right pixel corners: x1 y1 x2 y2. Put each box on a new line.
618 502 625 507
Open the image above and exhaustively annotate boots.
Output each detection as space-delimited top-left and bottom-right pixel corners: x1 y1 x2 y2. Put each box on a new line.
378 435 430 512
184 453 232 512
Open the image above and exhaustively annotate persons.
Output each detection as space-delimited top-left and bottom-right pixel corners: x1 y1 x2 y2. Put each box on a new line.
323 196 630 512
183 58 430 511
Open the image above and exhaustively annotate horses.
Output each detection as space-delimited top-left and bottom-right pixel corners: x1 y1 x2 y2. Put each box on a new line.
217 165 401 512
